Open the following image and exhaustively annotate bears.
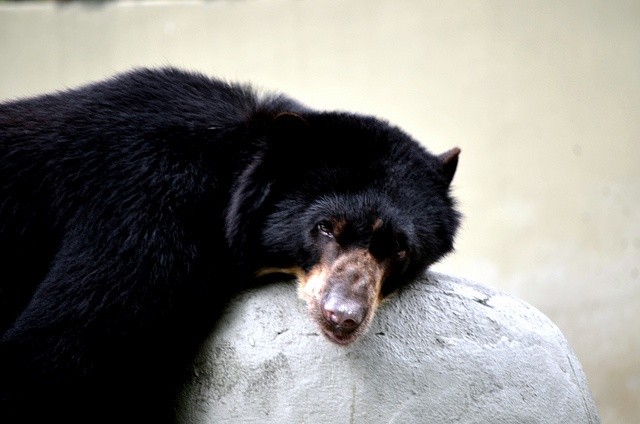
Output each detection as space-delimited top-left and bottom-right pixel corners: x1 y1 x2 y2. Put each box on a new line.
0 64 466 424
